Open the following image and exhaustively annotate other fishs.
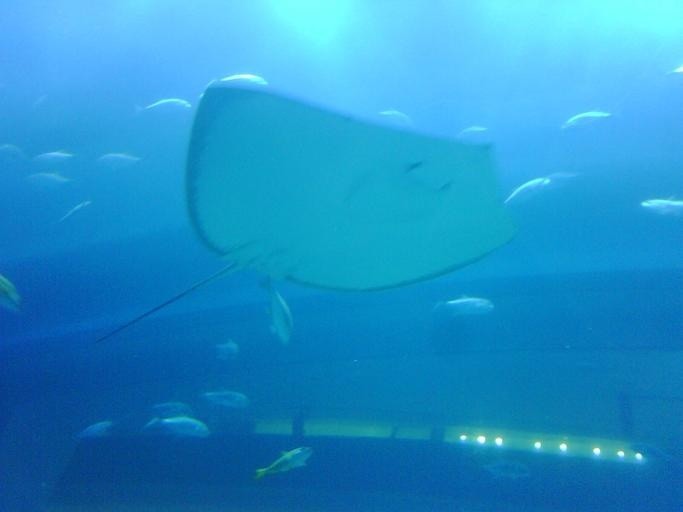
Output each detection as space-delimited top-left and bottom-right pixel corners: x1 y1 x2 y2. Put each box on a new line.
0 151 314 480
